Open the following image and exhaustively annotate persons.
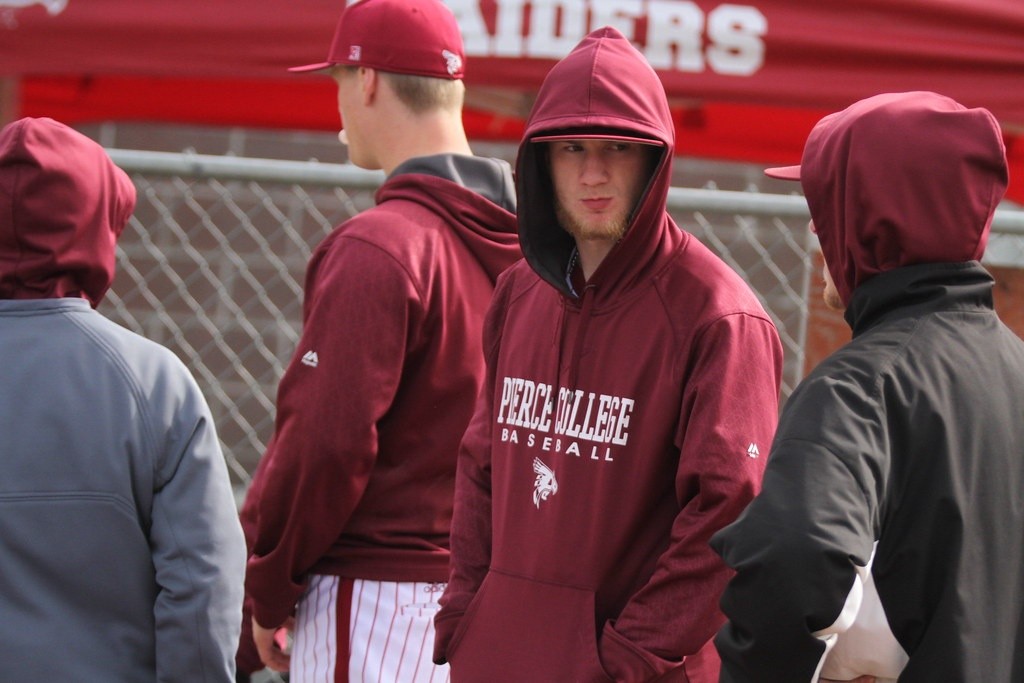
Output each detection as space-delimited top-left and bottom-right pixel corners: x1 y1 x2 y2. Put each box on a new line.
707 89 1023 683
0 116 247 682
432 26 788 683
240 0 526 683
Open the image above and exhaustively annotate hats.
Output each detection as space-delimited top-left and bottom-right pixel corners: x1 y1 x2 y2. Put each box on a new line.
529 128 665 147
764 165 801 181
287 0 465 79
